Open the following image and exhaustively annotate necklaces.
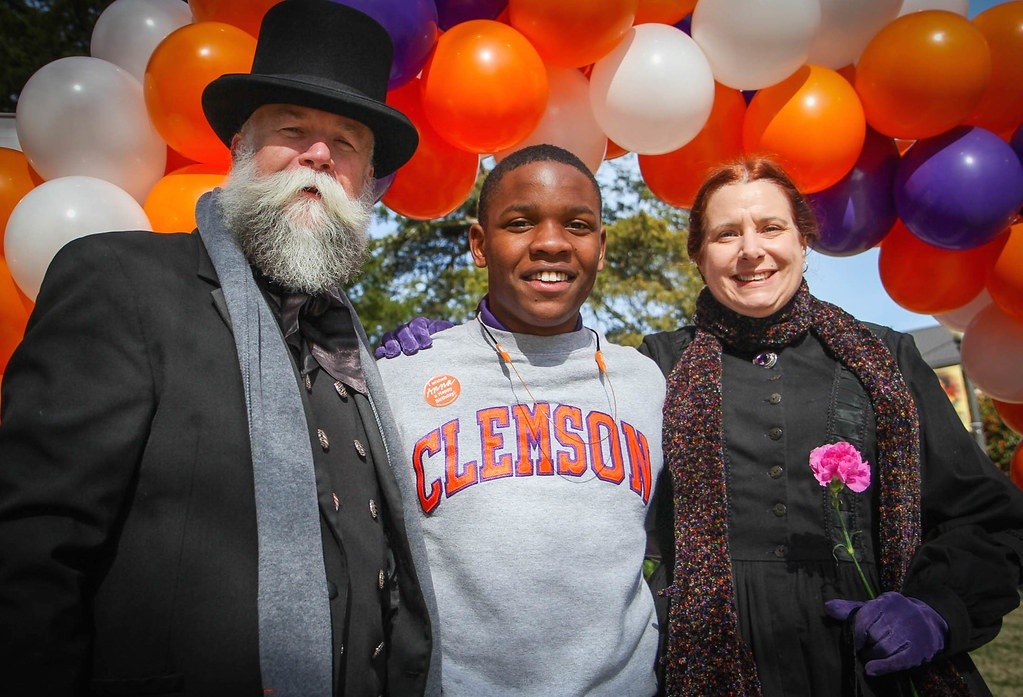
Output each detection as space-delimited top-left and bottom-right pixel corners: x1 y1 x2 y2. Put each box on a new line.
476 316 617 445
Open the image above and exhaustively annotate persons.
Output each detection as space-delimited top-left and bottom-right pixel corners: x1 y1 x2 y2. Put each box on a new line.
372 143 667 697
372 155 1023 697
0 0 444 697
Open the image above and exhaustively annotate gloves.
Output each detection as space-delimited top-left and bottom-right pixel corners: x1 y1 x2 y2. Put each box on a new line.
823 591 950 678
374 316 455 360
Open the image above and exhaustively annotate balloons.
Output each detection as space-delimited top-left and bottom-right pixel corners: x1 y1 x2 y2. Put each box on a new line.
0 0 1023 492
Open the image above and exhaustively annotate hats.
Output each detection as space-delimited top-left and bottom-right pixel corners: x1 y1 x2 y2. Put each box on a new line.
201 0 420 179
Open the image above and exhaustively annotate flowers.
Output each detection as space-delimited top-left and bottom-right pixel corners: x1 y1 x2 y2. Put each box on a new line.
808 441 922 697
